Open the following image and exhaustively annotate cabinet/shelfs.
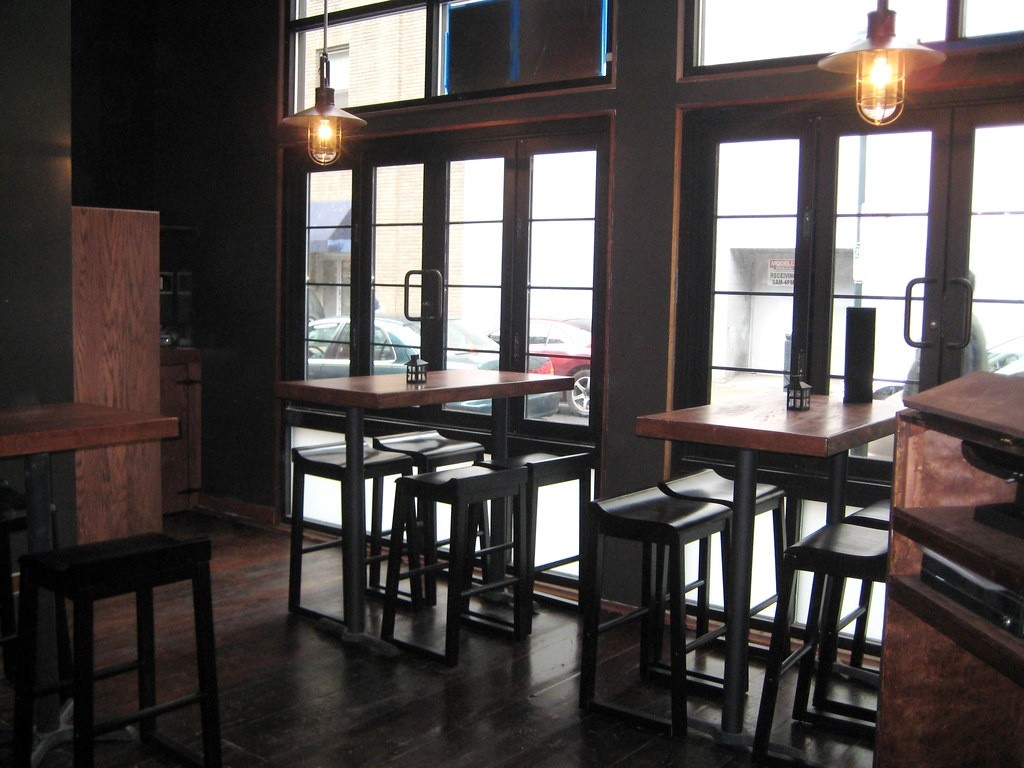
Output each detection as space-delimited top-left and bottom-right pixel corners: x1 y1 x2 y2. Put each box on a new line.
869 370 1024 768
67 204 168 545
161 347 201 513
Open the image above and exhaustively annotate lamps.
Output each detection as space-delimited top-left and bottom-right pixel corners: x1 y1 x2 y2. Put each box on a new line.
283 0 370 168
816 0 948 126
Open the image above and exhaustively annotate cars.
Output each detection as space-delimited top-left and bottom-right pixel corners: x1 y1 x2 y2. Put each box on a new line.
305 318 560 420
468 315 593 413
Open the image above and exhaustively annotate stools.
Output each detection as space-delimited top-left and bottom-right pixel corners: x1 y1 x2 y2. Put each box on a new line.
464 450 592 635
12 530 223 768
812 498 889 723
377 429 490 604
0 484 75 710
580 486 733 733
288 440 423 631
376 465 529 665
656 467 791 694
752 521 889 768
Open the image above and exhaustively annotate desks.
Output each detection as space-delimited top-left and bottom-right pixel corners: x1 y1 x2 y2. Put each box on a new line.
0 398 182 768
633 393 906 768
273 369 577 656
